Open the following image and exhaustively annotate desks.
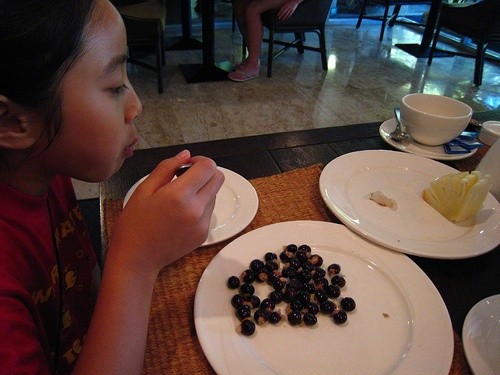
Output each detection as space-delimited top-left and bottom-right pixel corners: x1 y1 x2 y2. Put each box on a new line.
100 110 500 375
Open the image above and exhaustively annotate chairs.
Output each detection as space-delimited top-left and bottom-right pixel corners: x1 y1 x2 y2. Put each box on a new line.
115 0 166 94
242 0 333 79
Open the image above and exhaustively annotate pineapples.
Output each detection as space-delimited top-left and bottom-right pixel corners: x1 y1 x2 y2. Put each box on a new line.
422 170 492 223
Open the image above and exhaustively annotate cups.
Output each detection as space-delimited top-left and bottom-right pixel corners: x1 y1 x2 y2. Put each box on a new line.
399 93 473 147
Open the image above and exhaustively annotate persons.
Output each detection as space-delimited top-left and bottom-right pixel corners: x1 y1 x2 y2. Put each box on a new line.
0 0 225 375
228 0 303 82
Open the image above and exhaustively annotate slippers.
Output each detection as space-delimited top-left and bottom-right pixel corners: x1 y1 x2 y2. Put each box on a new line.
228 69 260 81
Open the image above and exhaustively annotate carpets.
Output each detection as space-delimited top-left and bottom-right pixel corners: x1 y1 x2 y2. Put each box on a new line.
361 15 500 61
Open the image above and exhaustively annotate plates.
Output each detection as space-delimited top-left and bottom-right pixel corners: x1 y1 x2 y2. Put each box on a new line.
121 164 259 247
318 148 500 259
379 116 480 161
463 294 500 375
194 219 455 375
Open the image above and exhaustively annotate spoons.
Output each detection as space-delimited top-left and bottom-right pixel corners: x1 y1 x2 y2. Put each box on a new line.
388 107 410 141
